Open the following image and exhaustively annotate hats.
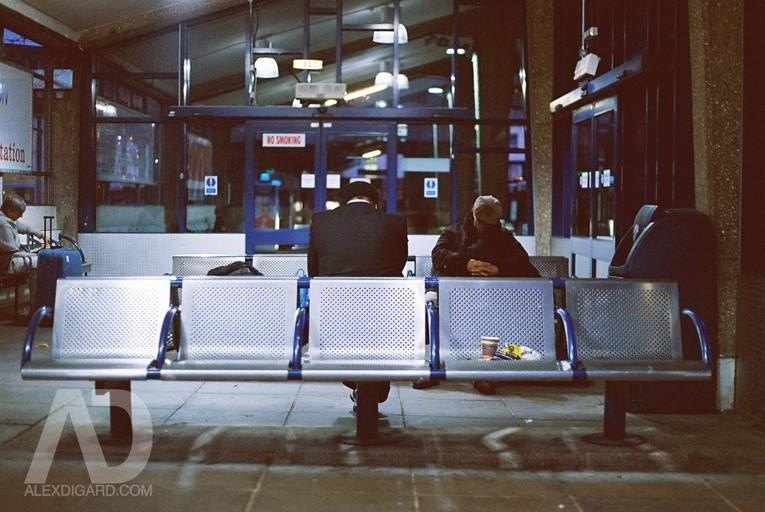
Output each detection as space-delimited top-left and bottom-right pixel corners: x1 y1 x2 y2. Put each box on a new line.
339 181 379 206
472 194 504 226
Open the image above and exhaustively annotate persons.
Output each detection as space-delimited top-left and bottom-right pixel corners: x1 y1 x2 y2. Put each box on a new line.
255 203 275 229
412 196 543 395
0 193 52 279
307 180 409 277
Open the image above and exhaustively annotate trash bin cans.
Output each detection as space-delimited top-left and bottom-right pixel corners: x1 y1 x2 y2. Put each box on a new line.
607 203 718 414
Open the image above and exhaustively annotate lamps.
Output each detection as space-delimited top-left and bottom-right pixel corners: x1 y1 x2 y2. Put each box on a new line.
373 60 409 89
373 7 408 45
254 42 279 79
361 147 382 159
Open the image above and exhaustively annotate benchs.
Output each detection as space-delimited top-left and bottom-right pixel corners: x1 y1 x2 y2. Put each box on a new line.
0 261 93 326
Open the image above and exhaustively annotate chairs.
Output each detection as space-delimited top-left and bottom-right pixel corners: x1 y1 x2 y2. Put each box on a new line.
20 254 713 439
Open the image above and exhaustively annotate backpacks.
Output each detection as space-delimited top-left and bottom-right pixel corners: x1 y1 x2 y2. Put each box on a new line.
207 261 263 275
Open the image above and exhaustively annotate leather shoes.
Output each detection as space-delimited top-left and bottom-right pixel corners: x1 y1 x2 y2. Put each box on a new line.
349 390 393 421
412 378 442 389
473 379 496 394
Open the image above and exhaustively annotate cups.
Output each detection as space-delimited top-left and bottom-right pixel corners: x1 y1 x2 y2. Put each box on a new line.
481 337 499 361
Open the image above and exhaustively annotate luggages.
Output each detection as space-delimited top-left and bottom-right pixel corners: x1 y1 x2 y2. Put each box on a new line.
35 216 83 327
